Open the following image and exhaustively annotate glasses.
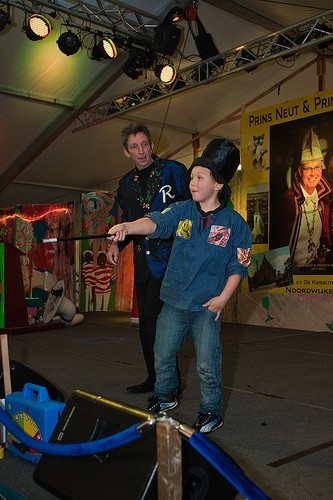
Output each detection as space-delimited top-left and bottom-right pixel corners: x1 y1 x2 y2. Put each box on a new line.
300 165 323 171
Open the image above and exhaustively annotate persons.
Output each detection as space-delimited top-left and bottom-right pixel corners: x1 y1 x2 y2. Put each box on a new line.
106 121 193 394
269 147 333 271
106 138 251 434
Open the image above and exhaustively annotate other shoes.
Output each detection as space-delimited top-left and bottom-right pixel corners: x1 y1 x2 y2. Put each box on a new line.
128 378 156 394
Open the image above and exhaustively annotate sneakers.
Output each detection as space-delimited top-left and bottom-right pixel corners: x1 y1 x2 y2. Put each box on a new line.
143 395 178 413
191 412 224 434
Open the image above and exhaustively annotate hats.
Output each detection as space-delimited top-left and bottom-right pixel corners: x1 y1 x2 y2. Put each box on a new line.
297 127 326 170
184 137 241 205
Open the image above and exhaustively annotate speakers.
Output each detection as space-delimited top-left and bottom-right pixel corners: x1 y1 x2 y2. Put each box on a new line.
34 389 244 500
0 358 64 402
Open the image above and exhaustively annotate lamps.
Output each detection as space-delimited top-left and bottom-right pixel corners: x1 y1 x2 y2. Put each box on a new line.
238 48 260 72
278 23 333 60
57 24 82 57
22 11 51 41
90 7 185 125
192 18 225 79
